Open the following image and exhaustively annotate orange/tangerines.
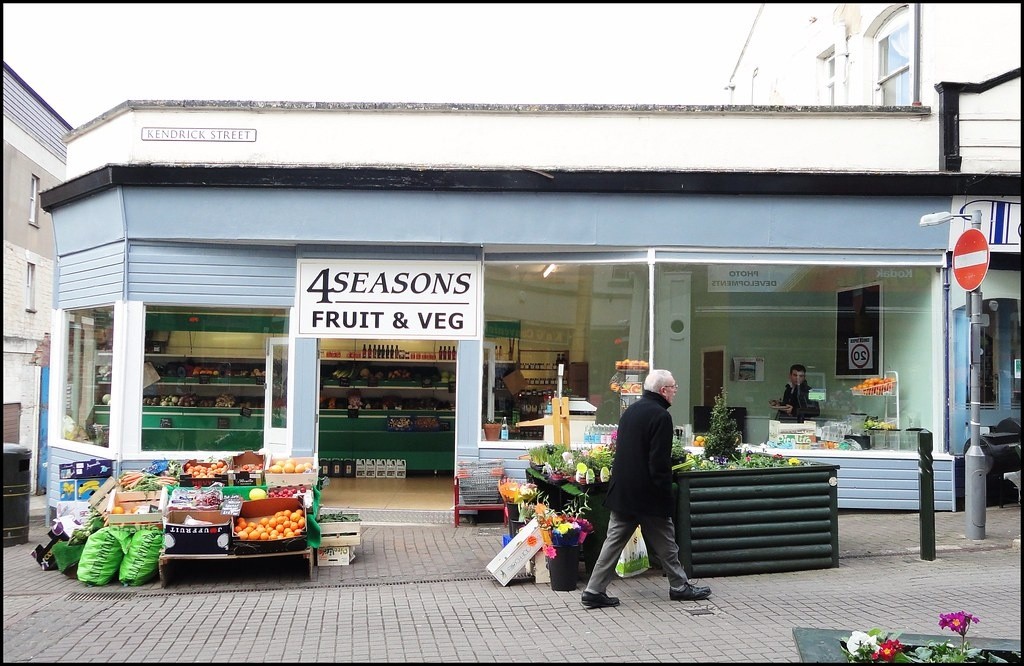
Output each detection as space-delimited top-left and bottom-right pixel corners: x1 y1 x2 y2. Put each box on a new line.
852 376 896 396
236 509 306 540
112 507 124 514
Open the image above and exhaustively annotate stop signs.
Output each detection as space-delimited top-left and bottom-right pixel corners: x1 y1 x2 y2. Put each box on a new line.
952 227 991 291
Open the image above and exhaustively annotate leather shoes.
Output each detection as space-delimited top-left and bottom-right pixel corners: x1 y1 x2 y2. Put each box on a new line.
670 583 711 601
581 591 620 607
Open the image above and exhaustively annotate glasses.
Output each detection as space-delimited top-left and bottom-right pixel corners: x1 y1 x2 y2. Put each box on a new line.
665 384 678 390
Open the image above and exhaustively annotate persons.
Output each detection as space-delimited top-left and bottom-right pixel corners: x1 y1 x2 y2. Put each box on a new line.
769 364 820 424
580 369 713 606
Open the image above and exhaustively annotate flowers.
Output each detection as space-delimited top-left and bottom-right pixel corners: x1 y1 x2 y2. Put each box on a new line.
840 610 982 663
498 387 813 545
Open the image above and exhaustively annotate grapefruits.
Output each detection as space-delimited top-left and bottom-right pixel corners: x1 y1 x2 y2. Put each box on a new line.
270 459 313 473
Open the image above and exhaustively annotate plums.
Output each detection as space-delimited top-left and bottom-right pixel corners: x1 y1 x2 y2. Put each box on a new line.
269 487 306 498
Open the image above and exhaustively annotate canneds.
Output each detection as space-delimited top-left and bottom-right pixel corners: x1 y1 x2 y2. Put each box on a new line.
326 349 341 358
410 351 436 359
519 362 567 395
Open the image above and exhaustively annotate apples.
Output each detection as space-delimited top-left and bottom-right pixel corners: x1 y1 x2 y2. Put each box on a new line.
865 420 896 430
193 365 220 376
185 461 227 478
251 369 266 377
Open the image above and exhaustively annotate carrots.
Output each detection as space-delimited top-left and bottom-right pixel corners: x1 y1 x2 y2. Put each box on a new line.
119 474 176 488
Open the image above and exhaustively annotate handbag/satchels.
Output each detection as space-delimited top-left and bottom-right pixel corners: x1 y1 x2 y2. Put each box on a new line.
615 524 650 578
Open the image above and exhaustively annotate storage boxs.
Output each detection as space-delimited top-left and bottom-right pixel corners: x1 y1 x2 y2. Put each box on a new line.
318 513 361 546
57 502 91 524
318 546 349 566
31 516 86 570
60 478 107 500
59 458 113 478
268 485 313 513
168 489 222 510
486 510 558 586
179 459 228 487
162 510 232 555
266 452 318 486
227 450 266 486
231 498 308 554
107 488 167 531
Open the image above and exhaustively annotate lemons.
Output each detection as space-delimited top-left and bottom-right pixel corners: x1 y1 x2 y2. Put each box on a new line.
694 434 707 446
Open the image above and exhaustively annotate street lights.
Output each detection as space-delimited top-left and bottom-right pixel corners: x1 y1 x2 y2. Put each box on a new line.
918 209 987 540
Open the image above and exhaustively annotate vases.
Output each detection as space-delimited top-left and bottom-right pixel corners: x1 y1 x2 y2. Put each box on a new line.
507 504 525 538
549 546 580 591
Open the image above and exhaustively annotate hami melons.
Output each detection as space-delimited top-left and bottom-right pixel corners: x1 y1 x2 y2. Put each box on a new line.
249 488 267 500
